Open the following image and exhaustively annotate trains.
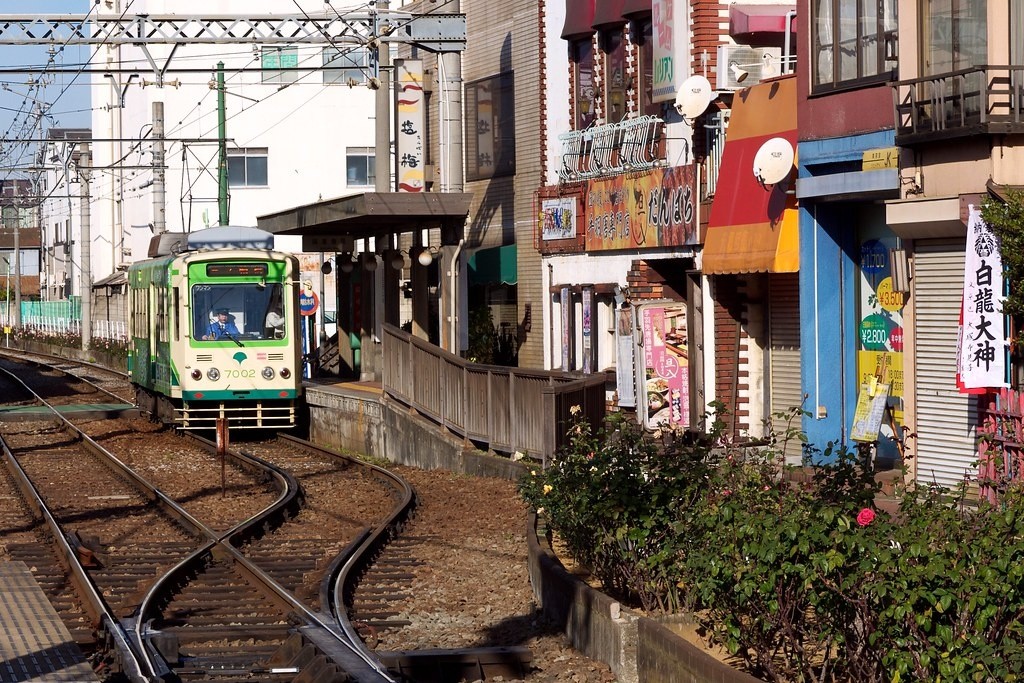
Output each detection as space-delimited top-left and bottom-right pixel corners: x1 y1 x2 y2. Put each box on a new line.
126 224 314 435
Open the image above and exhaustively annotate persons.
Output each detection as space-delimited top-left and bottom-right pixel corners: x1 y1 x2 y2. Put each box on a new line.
201 308 239 340
265 307 285 338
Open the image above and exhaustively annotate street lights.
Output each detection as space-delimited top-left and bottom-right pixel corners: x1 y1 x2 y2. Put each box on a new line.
2 256 10 326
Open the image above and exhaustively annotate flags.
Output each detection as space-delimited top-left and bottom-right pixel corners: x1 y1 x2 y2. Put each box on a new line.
955 203 1007 397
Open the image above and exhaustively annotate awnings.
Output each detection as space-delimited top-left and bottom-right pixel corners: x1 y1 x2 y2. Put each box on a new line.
468 245 517 286
702 77 800 274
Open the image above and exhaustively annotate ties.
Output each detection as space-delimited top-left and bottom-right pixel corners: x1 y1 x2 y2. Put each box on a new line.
222 325 224 331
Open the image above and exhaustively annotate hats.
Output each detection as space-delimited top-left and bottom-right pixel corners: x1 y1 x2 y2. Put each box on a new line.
216 308 230 315
